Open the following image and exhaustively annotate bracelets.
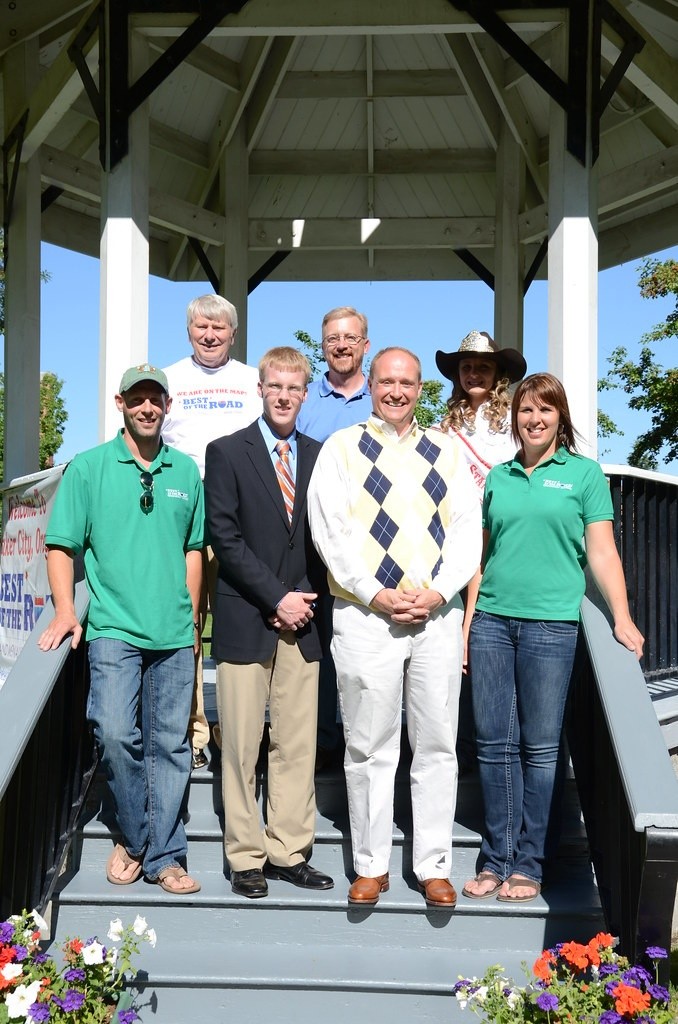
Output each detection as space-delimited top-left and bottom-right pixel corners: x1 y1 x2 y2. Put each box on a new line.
194 621 200 629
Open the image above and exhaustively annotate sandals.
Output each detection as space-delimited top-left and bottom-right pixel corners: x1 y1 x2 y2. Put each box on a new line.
462 870 505 898
496 876 541 902
106 840 141 884
155 865 201 893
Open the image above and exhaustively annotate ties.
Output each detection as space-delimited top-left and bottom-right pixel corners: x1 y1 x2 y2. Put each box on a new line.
274 439 295 526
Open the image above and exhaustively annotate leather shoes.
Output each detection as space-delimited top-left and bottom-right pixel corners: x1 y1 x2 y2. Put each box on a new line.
230 867 268 897
262 859 334 889
348 872 389 903
417 876 457 907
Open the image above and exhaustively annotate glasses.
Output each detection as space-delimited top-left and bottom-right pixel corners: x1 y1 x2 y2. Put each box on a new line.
139 472 153 509
322 334 366 345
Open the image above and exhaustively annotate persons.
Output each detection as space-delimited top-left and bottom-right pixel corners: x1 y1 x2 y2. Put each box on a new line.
429 332 527 775
306 347 483 907
37 363 205 894
462 373 644 902
293 307 373 773
204 346 334 898
160 295 265 768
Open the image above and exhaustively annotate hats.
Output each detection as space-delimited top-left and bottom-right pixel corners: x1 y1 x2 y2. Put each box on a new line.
119 364 169 397
435 331 527 388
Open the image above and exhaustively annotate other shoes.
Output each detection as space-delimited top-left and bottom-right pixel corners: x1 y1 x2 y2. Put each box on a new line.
192 747 208 766
